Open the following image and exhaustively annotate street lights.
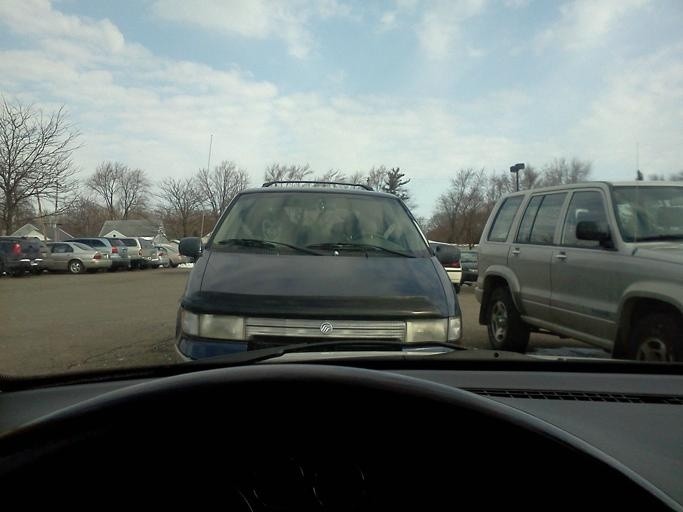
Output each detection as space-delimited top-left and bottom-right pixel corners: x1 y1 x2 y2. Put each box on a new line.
510 164 524 191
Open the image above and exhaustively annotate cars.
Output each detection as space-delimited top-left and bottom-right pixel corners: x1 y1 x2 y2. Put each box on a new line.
428 239 479 293
0 236 171 277
176 181 463 363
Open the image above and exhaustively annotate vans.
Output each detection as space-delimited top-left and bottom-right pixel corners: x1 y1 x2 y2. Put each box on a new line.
475 180 683 362
